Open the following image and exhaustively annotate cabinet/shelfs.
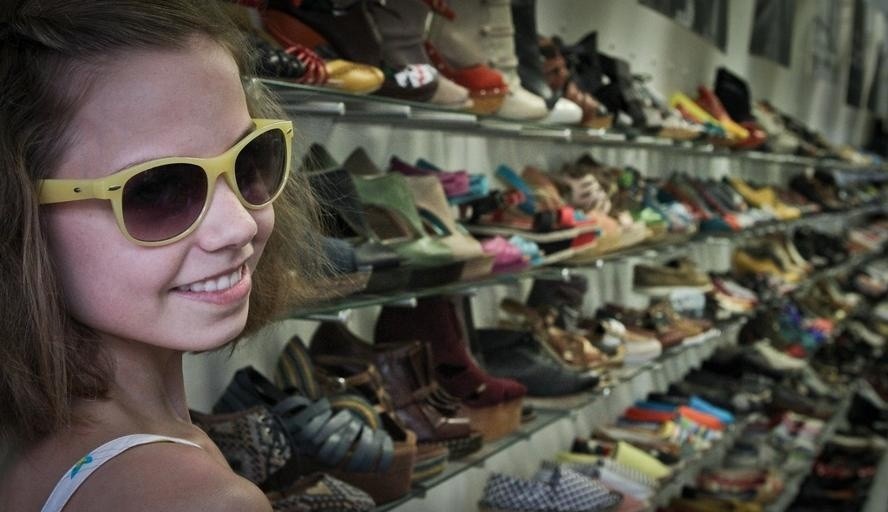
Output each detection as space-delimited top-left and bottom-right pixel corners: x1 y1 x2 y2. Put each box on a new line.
237 54 888 511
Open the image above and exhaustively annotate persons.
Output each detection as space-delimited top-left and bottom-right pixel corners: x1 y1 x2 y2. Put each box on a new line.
0 0 294 512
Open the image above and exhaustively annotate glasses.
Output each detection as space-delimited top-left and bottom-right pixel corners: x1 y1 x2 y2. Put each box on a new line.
32 117 294 245
